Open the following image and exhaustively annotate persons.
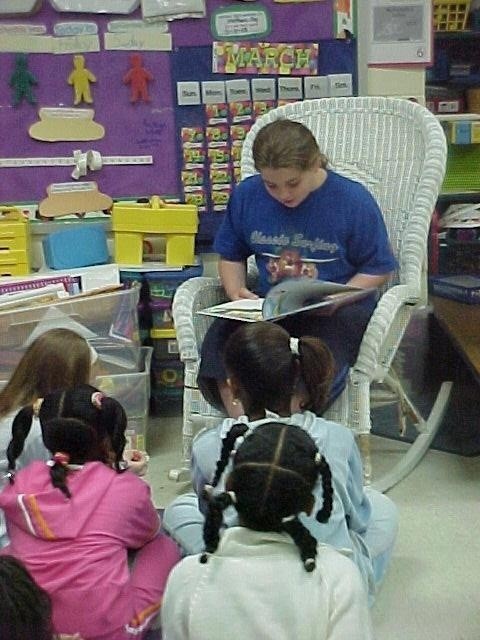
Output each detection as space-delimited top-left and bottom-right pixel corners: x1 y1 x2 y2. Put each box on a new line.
2 327 184 640
163 323 400 639
192 119 406 422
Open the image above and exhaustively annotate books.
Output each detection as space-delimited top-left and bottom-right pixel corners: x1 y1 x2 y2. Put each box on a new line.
195 271 359 324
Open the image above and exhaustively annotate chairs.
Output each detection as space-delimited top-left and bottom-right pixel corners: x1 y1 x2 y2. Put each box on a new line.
167 96 454 494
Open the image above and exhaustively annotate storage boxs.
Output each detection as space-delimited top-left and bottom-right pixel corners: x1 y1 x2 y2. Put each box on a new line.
0 281 143 374
120 265 203 415
0 346 153 452
